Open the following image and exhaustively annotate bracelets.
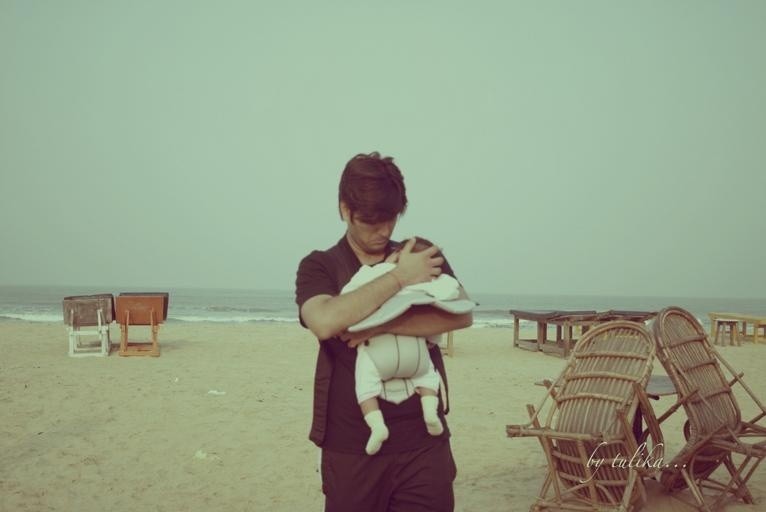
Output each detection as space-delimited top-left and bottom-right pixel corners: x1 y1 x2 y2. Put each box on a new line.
387 270 405 290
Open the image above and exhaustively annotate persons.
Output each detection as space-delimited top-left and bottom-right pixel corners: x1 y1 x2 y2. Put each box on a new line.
337 236 474 455
293 151 476 511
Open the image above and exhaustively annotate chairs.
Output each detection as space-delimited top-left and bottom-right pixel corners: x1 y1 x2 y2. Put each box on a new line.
502 305 766 511
